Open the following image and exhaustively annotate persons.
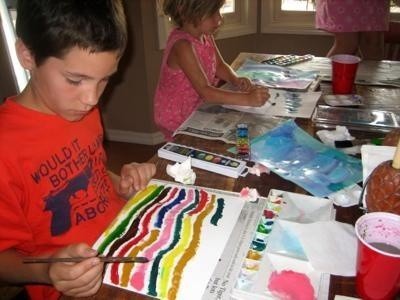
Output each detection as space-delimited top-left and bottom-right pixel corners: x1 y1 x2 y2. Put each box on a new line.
0 0 156 299
152 0 270 140
313 0 389 63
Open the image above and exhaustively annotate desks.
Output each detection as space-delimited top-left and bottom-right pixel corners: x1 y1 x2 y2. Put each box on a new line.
57 51 400 300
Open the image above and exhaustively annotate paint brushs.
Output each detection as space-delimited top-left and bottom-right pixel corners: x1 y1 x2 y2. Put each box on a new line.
22 258 149 264
335 137 384 148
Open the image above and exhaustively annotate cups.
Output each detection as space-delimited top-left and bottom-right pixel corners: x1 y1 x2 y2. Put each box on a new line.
330 54 362 95
353 209 400 300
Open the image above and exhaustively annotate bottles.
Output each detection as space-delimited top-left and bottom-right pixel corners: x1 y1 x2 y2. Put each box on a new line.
235 122 253 163
